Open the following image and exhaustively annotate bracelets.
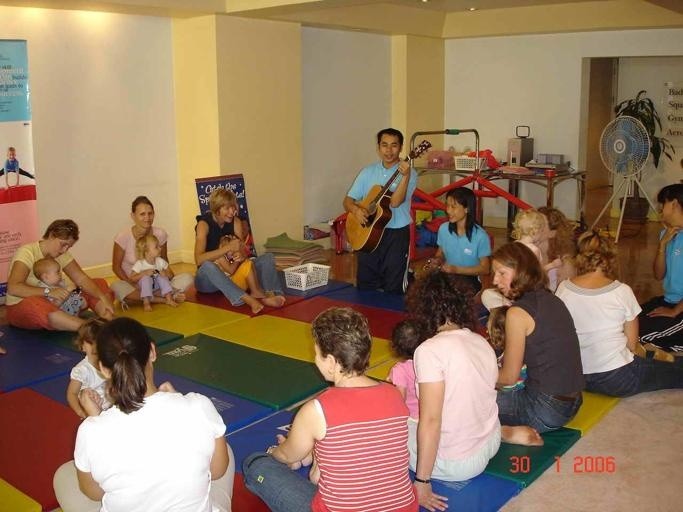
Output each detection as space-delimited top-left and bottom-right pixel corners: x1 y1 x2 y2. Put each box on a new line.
437 263 442 271
413 475 432 484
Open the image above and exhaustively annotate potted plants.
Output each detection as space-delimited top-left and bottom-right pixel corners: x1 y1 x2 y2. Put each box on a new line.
614 90 676 220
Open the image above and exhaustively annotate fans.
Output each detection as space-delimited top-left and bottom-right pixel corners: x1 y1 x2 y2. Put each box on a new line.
590 115 669 244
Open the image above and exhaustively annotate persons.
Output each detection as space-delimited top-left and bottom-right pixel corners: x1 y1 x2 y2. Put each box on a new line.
639 184 682 349
422 186 492 296
482 241 584 432
112 196 185 311
4 217 114 350
413 271 542 511
53 319 236 512
243 304 420 512
512 206 683 399
33 258 88 315
387 319 421 420
194 188 285 313
343 128 418 296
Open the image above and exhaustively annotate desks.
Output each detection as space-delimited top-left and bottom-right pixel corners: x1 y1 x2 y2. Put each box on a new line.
414 166 591 230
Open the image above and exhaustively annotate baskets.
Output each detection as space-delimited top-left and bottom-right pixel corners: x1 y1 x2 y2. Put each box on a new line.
455 155 488 170
282 263 331 292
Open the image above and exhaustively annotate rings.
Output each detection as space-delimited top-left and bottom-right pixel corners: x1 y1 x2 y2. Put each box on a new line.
428 259 430 263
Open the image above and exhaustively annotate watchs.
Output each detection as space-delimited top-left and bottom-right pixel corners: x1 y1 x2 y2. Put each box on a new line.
44 287 50 296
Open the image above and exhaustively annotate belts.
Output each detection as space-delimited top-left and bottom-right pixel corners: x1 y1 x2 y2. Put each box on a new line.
555 394 576 401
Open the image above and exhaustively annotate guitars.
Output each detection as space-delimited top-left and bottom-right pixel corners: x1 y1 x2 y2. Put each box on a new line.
345 140 432 253
